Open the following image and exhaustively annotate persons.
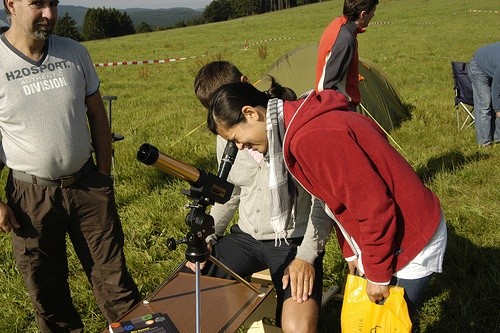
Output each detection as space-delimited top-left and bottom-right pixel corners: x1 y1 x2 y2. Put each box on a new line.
208 75 447 332
467 41 500 148
316 0 379 113
0 0 143 333
175 61 334 333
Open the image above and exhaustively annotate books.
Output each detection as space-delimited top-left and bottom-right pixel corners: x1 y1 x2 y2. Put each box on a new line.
109 312 179 333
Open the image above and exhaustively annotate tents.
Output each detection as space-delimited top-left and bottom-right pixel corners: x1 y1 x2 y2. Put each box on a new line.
254 40 412 137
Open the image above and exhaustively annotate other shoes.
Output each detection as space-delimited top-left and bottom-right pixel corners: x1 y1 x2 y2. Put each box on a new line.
495 143 500 147
480 143 493 149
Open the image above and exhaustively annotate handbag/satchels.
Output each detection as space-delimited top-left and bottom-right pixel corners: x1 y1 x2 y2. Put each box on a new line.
340 273 412 333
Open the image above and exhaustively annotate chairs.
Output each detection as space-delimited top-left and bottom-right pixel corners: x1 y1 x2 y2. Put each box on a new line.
451 61 475 132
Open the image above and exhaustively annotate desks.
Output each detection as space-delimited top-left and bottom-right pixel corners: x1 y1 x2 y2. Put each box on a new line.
101 272 274 333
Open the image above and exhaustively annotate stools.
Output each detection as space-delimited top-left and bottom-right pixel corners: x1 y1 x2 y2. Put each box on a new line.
243 268 282 333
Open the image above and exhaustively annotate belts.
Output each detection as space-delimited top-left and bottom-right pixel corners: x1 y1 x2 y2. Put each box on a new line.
9 152 94 188
